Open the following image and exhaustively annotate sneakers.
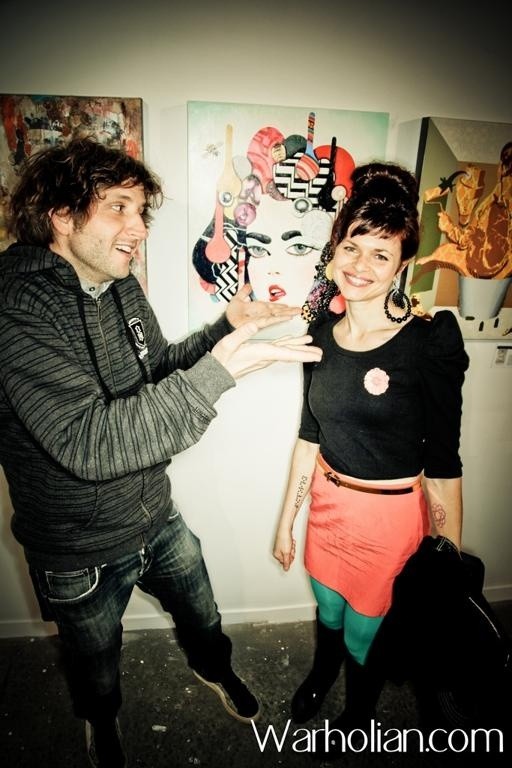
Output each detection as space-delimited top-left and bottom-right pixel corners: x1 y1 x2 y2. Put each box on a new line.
81 713 128 767
193 671 261 723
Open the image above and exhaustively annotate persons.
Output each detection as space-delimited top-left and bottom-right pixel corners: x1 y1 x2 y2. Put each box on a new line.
1 139 322 768
193 113 355 308
272 162 470 752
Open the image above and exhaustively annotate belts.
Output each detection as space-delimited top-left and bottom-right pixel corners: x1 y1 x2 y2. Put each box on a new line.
318 455 422 496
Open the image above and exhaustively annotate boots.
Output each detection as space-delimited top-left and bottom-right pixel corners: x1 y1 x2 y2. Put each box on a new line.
313 662 373 759
291 607 345 724
